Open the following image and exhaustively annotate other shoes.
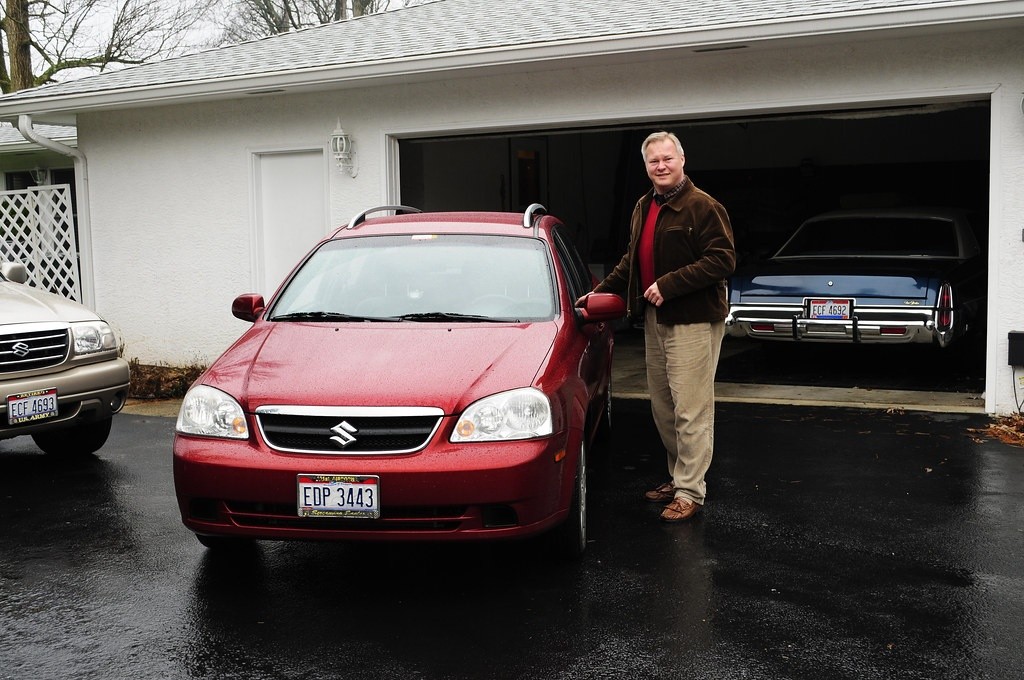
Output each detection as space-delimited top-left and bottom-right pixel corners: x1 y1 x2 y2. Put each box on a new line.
643 482 676 503
659 497 702 522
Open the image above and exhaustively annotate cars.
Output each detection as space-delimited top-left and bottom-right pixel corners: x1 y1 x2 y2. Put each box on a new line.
170 202 628 558
723 211 987 363
0 265 131 457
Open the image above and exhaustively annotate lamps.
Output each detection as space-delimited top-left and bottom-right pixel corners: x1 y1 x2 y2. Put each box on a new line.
30 167 47 185
330 116 358 178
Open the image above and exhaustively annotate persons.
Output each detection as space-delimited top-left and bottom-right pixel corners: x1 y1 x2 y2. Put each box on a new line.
574 132 736 519
